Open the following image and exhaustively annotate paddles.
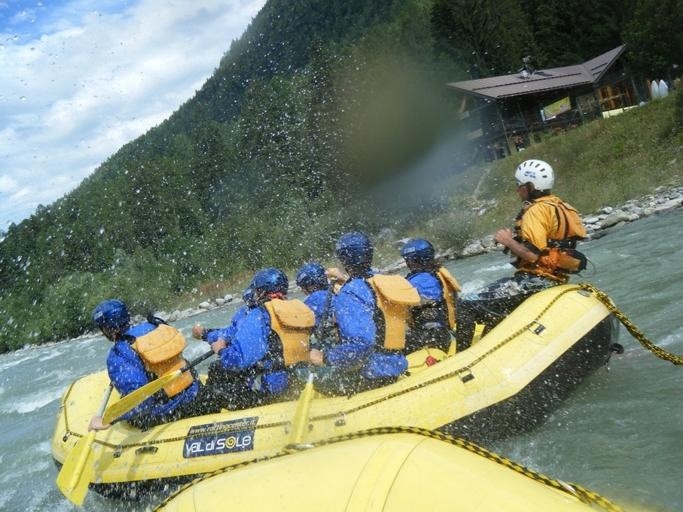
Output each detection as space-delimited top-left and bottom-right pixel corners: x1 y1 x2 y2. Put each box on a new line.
103 339 232 425
283 275 336 449
56 381 114 507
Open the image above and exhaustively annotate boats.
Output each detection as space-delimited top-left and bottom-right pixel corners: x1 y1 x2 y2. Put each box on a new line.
150 425 617 508
51 281 618 501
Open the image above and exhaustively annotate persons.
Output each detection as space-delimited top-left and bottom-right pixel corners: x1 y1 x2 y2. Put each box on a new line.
295 262 337 352
87 298 206 436
200 268 316 415
396 236 463 358
307 230 421 397
191 275 258 346
452 158 588 356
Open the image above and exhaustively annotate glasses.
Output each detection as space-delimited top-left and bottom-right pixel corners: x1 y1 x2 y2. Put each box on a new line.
515 182 525 188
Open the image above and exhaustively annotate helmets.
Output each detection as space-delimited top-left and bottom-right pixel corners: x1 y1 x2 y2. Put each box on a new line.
514 159 555 193
92 297 130 329
240 283 256 306
252 267 288 295
295 263 327 287
401 239 434 265
336 230 373 266
